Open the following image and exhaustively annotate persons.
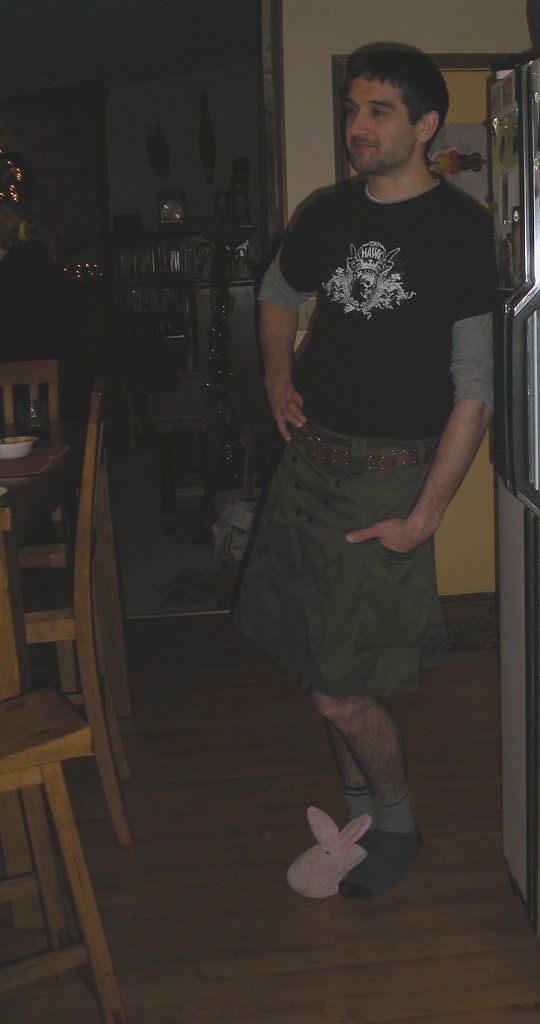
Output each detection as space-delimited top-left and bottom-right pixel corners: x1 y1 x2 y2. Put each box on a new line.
233 43 496 902
0 201 78 421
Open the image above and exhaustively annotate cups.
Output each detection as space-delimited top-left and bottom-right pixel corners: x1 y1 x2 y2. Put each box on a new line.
22 400 48 437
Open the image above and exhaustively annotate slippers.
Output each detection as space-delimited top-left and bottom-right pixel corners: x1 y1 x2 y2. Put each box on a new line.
286 804 372 899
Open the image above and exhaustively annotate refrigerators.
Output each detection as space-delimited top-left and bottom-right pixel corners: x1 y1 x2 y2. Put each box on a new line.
483 45 540 933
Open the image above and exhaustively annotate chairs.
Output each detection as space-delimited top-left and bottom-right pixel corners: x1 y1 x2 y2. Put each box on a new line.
0 359 136 1024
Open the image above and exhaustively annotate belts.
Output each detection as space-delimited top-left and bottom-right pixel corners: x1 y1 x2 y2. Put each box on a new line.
288 425 439 474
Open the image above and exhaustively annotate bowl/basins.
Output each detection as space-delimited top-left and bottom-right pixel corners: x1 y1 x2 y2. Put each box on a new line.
0 436 39 459
